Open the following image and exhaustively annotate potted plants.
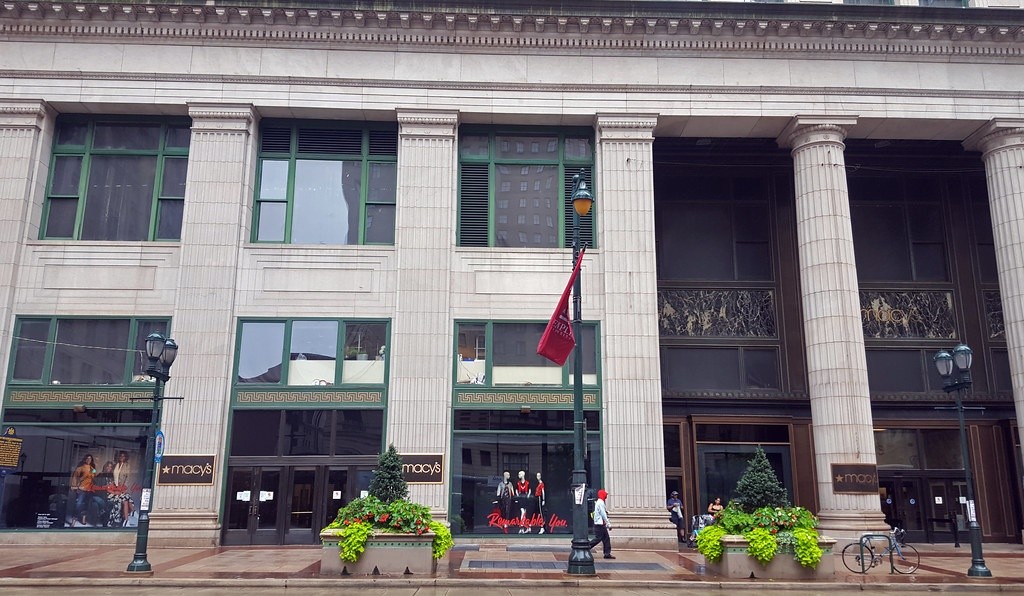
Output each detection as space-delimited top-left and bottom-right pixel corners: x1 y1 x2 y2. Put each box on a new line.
356 350 369 361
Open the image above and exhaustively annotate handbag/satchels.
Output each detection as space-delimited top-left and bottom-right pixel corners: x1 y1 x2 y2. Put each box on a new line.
590 511 608 521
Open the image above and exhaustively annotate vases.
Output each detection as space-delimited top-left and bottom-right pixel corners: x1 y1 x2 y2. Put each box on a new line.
320 528 438 575
703 535 837 581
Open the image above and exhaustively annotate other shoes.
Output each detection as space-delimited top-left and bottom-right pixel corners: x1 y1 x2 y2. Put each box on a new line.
130 511 139 519
122 519 131 527
681 535 686 543
604 555 616 559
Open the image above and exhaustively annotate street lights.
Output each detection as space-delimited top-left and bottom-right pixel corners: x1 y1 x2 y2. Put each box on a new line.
933 343 994 577
566 166 594 572
126 331 184 571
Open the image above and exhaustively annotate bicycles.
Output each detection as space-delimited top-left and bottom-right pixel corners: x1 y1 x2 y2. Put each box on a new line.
842 527 920 575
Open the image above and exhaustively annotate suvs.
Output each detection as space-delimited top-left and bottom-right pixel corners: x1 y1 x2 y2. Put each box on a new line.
453 476 536 527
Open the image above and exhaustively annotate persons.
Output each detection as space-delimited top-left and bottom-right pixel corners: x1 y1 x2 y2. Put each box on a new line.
667 491 686 543
708 498 723 518
515 471 531 534
525 472 546 535
91 461 115 527
590 489 616 559
496 472 514 534
70 454 97 528
113 451 136 528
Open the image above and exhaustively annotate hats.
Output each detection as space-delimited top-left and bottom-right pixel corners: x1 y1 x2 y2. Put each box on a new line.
672 491 678 495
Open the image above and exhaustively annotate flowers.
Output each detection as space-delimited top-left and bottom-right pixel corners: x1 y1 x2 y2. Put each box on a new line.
696 447 819 564
322 443 459 562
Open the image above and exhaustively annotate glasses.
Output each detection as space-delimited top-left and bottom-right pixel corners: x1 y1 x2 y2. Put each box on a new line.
673 495 677 496
